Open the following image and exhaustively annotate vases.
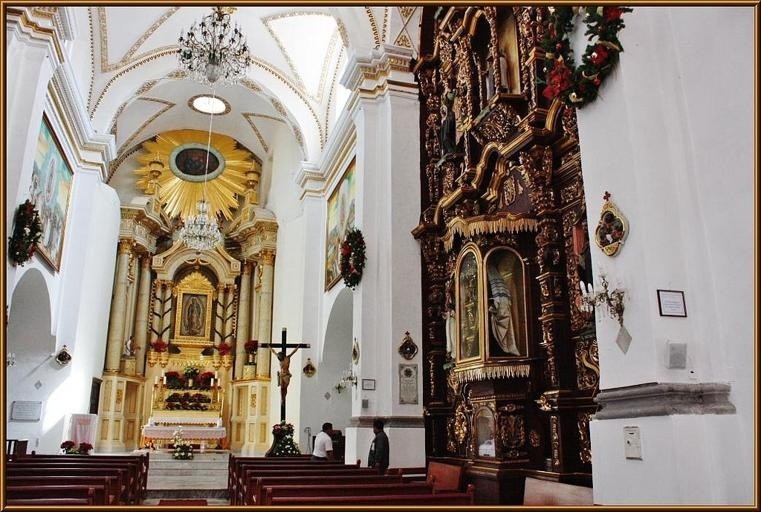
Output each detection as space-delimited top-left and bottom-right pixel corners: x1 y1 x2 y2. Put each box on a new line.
188 379 193 387
248 354 255 365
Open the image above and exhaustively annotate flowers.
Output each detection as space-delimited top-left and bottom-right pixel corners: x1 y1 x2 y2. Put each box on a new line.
171 430 193 459
183 366 200 378
245 340 258 354
59 440 93 454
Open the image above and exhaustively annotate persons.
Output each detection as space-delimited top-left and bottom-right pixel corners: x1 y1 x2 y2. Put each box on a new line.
184 297 202 333
311 422 335 460
266 343 300 400
125 335 136 355
367 419 390 469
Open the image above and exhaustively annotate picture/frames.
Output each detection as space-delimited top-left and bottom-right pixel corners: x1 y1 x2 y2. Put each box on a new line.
302 331 418 390
595 200 629 258
55 350 72 366
322 148 357 293
26 110 75 274
657 289 687 317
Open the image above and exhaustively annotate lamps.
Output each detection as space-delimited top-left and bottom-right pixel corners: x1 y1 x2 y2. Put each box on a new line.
579 274 624 328
6 352 16 367
179 85 223 267
172 5 255 81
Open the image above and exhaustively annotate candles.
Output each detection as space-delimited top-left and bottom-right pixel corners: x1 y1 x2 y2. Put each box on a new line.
210 371 221 386
155 369 166 385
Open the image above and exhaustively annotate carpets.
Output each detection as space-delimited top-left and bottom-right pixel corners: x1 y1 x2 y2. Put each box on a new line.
157 499 208 506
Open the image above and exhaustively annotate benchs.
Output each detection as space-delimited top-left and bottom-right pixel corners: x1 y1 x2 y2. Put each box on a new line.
226 453 480 512
4 452 150 508
